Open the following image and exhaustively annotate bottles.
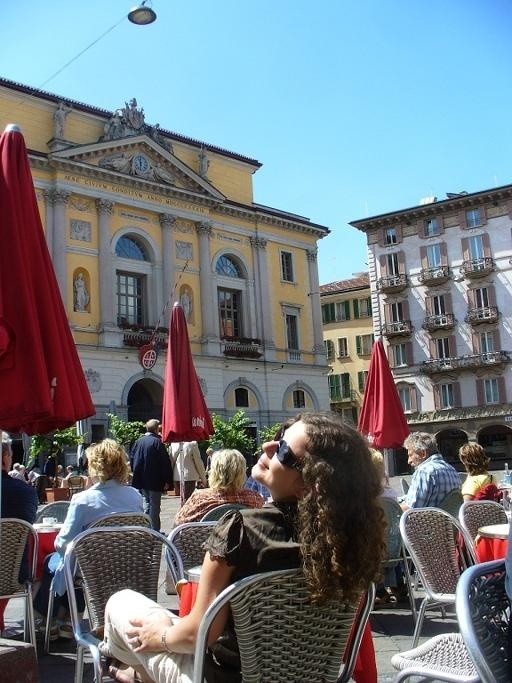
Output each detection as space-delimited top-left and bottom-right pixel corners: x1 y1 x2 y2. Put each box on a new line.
502 461 511 485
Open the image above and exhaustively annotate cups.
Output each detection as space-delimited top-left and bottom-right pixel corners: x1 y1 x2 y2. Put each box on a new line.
41 516 58 524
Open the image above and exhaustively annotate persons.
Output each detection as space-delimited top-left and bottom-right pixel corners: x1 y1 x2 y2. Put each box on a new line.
149 161 175 184
53 101 73 138
31 437 150 640
102 98 160 143
100 152 134 171
0 414 511 683
98 411 388 683
198 145 211 180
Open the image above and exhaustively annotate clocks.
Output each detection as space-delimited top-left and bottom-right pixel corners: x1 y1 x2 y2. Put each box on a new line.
134 155 151 174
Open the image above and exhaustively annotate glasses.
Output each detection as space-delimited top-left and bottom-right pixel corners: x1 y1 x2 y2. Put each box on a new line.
274 424 305 475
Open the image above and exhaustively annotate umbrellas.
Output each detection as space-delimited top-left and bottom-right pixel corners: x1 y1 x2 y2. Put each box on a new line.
355 342 408 482
162 300 211 505
0 123 96 438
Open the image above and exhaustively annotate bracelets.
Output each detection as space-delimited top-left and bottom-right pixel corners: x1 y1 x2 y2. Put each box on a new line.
160 626 172 654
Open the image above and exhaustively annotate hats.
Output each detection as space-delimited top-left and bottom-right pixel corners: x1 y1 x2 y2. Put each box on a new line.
145 418 160 432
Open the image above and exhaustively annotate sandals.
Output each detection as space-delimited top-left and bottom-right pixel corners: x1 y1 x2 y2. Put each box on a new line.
375 592 393 609
33 619 57 641
106 657 141 683
54 620 74 639
